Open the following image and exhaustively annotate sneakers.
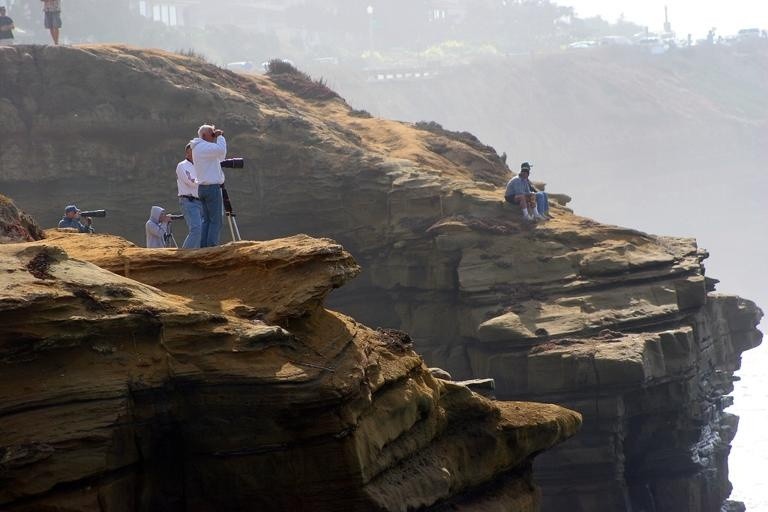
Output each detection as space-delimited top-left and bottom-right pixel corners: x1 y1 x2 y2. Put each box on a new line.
524 214 554 221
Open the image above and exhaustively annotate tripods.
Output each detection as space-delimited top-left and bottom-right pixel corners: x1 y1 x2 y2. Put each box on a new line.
219 183 241 241
164 221 178 248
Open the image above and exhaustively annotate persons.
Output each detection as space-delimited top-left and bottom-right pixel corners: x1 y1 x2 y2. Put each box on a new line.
145 207 174 249
504 162 554 223
58 206 97 233
0 6 15 43
43 0 61 46
176 125 226 249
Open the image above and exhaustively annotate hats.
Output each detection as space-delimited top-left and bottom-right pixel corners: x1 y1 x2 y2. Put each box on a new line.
521 162 533 172
65 206 80 212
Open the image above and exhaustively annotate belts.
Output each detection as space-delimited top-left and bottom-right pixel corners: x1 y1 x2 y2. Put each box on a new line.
182 195 199 200
